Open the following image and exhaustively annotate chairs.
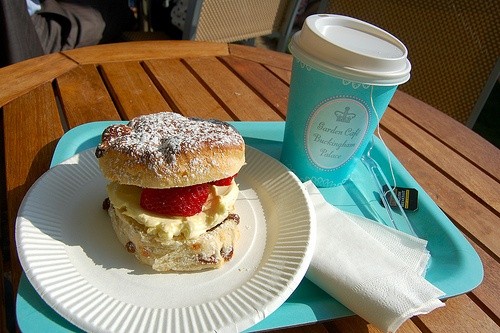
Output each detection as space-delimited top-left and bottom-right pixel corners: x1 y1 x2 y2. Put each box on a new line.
150 0 308 53
318 0 500 130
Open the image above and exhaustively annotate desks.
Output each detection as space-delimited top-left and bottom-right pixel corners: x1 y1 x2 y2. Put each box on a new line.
0 40 500 333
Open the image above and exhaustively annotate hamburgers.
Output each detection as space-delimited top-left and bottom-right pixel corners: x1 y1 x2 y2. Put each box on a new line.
95 111 245 271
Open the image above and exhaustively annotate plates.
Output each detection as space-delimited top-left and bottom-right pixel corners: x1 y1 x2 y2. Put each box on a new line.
14 144 315 333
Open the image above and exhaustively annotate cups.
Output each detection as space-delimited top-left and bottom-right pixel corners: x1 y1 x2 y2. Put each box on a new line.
280 13 412 187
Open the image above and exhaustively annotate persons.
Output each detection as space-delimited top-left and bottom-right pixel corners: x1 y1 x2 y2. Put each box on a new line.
26 0 105 55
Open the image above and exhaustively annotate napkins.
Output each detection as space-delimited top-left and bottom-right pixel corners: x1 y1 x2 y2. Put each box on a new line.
302 180 446 333
